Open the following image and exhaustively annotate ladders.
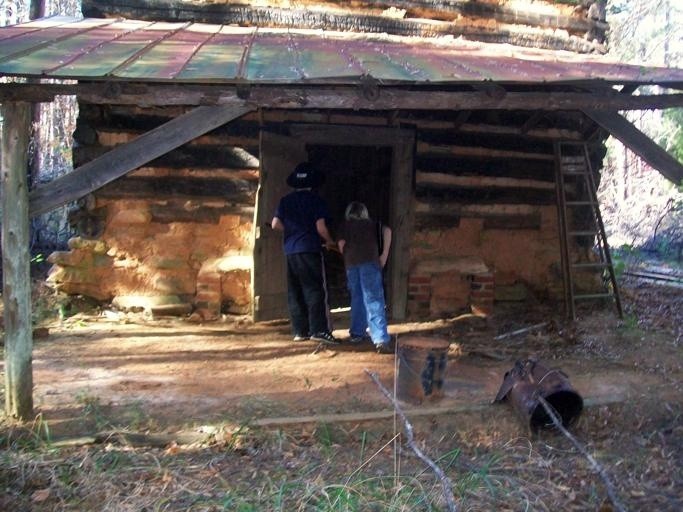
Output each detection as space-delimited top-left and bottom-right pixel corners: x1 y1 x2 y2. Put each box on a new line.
555 138 638 324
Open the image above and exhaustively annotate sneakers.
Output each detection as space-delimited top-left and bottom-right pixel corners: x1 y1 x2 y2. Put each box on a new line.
294 330 342 345
350 332 369 343
375 343 393 353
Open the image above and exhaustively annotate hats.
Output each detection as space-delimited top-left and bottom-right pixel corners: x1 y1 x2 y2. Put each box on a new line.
285 161 324 188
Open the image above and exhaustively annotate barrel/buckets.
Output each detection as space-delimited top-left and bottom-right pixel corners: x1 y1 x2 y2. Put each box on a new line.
396 334 449 406
508 360 583 438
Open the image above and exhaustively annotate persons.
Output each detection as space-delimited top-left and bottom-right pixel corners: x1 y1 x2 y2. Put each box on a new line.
336 202 392 353
271 160 342 344
